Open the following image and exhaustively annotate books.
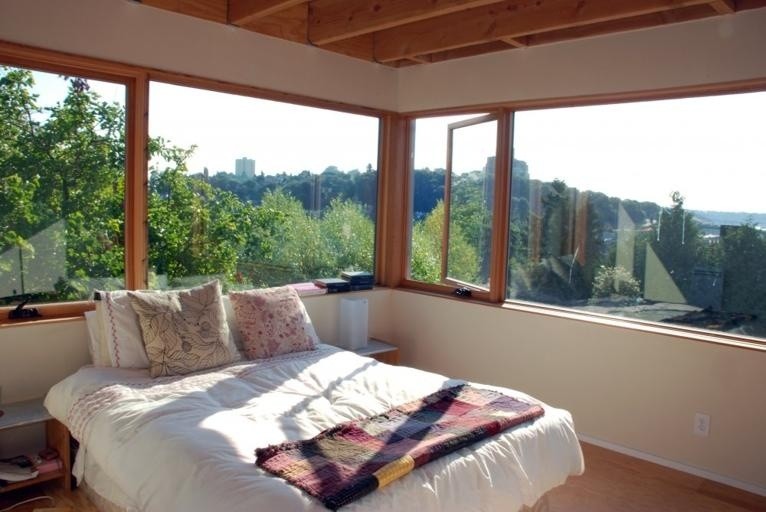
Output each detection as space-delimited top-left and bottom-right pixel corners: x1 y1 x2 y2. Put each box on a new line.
0 453 38 482
290 271 375 297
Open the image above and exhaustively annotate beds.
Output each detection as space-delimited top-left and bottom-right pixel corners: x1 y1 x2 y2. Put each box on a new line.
44 287 585 512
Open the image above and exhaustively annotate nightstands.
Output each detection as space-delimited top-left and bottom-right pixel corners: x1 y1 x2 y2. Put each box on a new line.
339 337 400 366
0 399 73 497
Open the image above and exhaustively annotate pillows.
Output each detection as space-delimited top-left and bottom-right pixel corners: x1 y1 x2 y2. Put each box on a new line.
227 285 320 363
128 278 243 380
83 309 112 368
94 290 166 370
